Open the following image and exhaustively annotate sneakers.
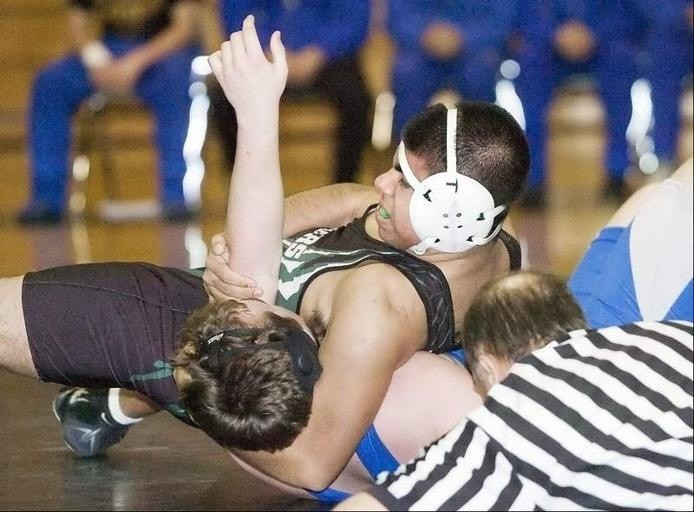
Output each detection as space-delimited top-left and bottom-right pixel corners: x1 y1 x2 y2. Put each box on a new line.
53 385 132 458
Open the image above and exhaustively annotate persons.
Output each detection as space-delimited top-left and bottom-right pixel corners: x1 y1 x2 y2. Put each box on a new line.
0 102 524 497
15 1 372 229
327 267 693 512
385 1 692 211
174 14 694 503
